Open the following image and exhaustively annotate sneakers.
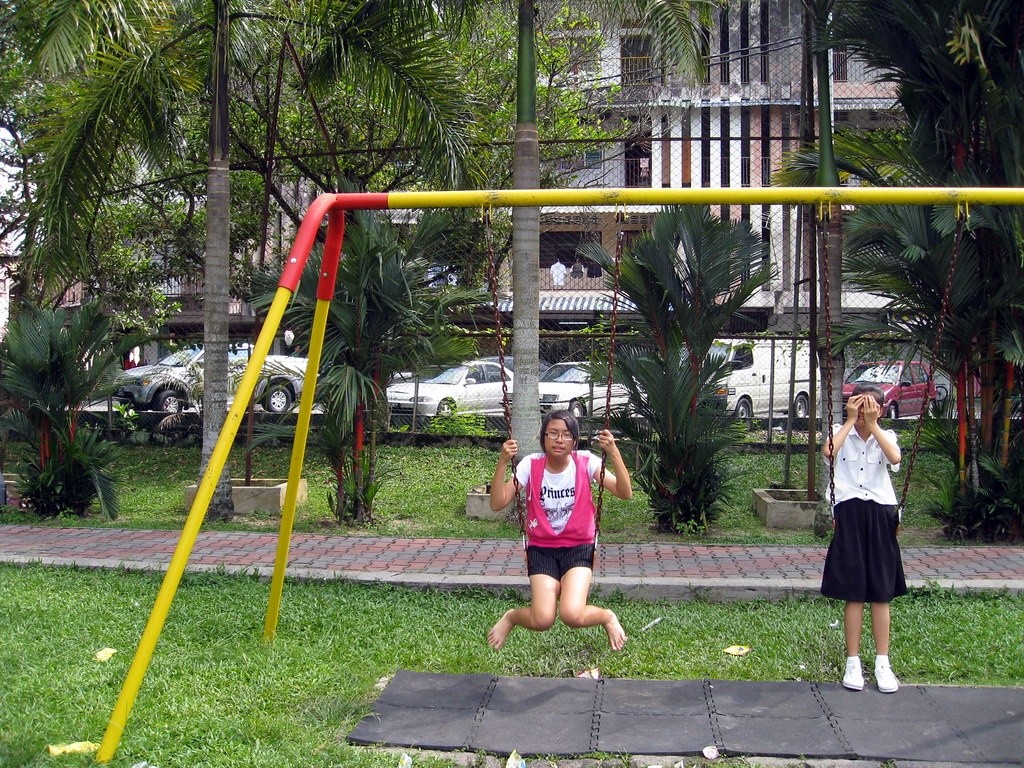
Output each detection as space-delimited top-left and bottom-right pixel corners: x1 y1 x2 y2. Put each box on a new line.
842 662 864 690
874 664 900 693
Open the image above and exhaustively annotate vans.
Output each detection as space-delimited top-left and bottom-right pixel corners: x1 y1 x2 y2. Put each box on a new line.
680 337 821 432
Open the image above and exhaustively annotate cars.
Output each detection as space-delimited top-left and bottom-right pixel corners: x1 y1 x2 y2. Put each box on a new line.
843 360 937 419
498 361 654 435
386 354 559 430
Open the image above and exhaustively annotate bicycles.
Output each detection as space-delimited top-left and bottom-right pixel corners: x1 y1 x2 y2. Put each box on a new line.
934 383 948 401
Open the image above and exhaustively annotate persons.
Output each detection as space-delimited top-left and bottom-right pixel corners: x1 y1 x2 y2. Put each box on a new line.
820 385 908 693
488 410 632 650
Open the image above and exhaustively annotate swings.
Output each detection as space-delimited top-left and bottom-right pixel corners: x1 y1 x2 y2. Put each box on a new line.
821 212 968 539
481 210 626 576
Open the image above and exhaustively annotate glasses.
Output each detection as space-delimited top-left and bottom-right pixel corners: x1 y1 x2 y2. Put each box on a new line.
544 429 576 441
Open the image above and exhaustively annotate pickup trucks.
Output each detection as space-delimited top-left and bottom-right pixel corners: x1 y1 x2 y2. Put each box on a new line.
110 340 309 414
